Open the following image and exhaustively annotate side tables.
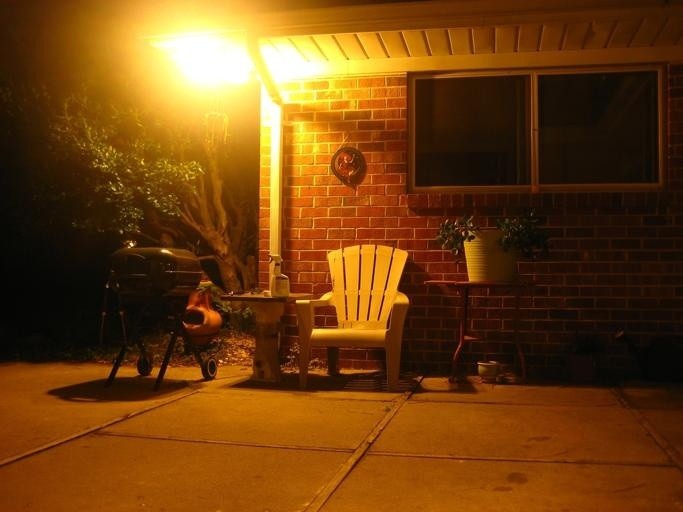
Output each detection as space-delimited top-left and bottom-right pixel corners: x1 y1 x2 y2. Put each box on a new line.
219 290 313 386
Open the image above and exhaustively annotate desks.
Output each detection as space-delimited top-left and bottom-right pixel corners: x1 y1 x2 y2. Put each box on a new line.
421 279 532 385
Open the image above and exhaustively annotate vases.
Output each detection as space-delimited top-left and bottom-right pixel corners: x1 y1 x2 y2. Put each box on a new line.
477 361 498 383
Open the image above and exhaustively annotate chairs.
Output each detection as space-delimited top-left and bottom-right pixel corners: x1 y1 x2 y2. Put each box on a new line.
294 244 409 390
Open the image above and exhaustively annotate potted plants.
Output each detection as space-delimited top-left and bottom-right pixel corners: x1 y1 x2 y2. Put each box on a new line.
435 209 549 281
